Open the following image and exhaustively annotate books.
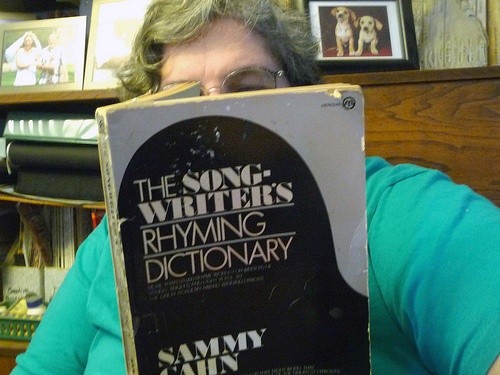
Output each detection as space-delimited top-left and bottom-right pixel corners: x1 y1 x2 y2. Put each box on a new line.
95 80 371 375
10 202 95 271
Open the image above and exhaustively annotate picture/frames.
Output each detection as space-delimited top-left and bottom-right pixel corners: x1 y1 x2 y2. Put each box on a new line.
84 0 152 90
1 15 87 92
303 0 420 75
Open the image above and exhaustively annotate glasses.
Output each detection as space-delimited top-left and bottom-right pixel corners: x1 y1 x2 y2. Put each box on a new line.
154 67 288 96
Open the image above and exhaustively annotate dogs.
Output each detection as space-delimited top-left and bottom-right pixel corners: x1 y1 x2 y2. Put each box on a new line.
331 6 383 57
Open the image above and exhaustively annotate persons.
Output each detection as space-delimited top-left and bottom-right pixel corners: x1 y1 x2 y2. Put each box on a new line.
10 0 500 375
14 32 43 86
36 31 72 84
